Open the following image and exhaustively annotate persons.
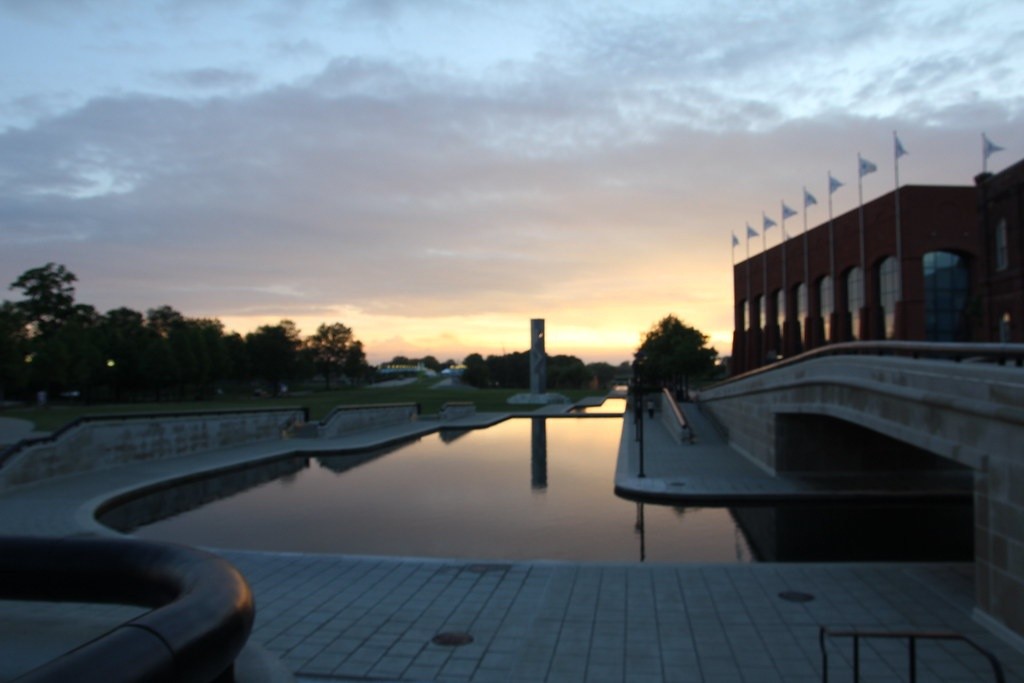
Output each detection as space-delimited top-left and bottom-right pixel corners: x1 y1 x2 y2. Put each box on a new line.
689 387 696 403
648 399 655 418
